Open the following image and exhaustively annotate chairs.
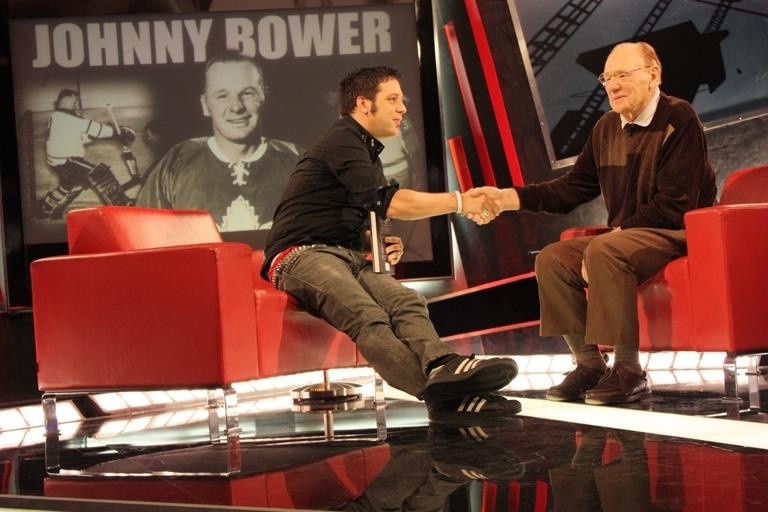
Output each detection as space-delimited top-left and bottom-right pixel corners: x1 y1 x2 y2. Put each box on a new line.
30 208 388 478
560 165 767 398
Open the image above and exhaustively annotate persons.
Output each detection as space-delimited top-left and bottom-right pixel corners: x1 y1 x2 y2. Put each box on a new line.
461 43 717 404
36 88 138 220
547 395 656 511
131 52 308 233
338 416 530 511
260 65 521 420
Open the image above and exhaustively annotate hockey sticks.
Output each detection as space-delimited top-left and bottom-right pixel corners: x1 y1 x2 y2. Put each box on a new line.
106 102 142 191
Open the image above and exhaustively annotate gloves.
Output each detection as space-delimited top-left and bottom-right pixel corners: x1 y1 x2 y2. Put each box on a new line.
112 124 136 148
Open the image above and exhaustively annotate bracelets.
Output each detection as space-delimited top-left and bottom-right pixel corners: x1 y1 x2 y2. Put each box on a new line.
454 190 462 214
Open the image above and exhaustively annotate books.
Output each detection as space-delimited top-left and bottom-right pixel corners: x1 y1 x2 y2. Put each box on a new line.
369 211 396 275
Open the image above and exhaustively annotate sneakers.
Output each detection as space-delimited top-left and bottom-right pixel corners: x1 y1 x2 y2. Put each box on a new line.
429 410 524 444
425 355 519 399
437 438 525 482
425 393 522 419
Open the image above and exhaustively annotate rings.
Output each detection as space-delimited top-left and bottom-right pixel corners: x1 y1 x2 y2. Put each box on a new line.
483 208 488 217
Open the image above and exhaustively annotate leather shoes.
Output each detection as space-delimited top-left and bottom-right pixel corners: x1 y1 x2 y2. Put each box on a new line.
583 367 653 406
545 367 610 403
